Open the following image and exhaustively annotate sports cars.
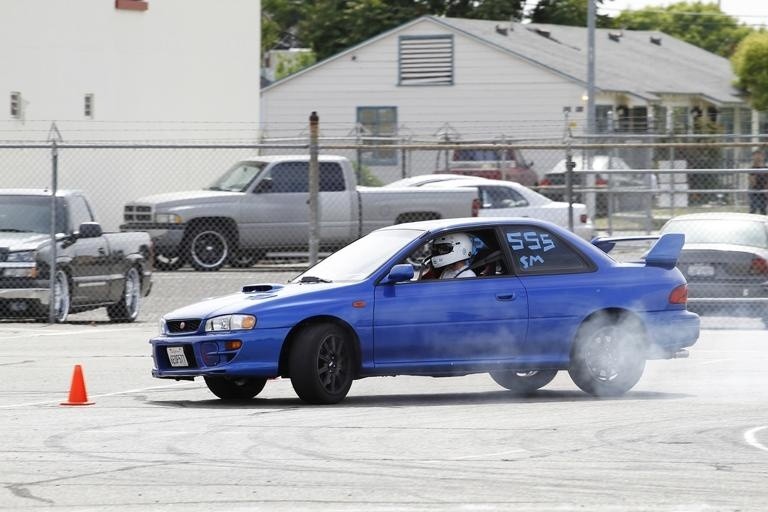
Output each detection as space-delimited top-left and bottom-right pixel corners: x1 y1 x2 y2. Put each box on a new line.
149 216 700 403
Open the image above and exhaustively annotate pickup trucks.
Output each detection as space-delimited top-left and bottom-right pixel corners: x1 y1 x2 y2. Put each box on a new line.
117 154 480 274
0 187 154 325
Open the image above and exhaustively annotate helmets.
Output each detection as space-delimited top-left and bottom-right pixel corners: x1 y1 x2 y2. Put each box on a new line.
429 232 473 270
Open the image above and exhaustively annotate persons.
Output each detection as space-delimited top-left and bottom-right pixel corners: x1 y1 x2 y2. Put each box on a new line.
748 151 768 215
431 232 478 280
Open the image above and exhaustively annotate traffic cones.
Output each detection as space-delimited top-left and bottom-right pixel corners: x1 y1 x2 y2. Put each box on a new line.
61 364 96 405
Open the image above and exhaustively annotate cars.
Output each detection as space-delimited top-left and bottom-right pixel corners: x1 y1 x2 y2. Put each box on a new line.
383 172 597 243
383 141 658 243
659 212 768 326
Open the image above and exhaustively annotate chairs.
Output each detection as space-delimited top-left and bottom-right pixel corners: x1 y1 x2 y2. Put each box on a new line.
471 248 495 277
